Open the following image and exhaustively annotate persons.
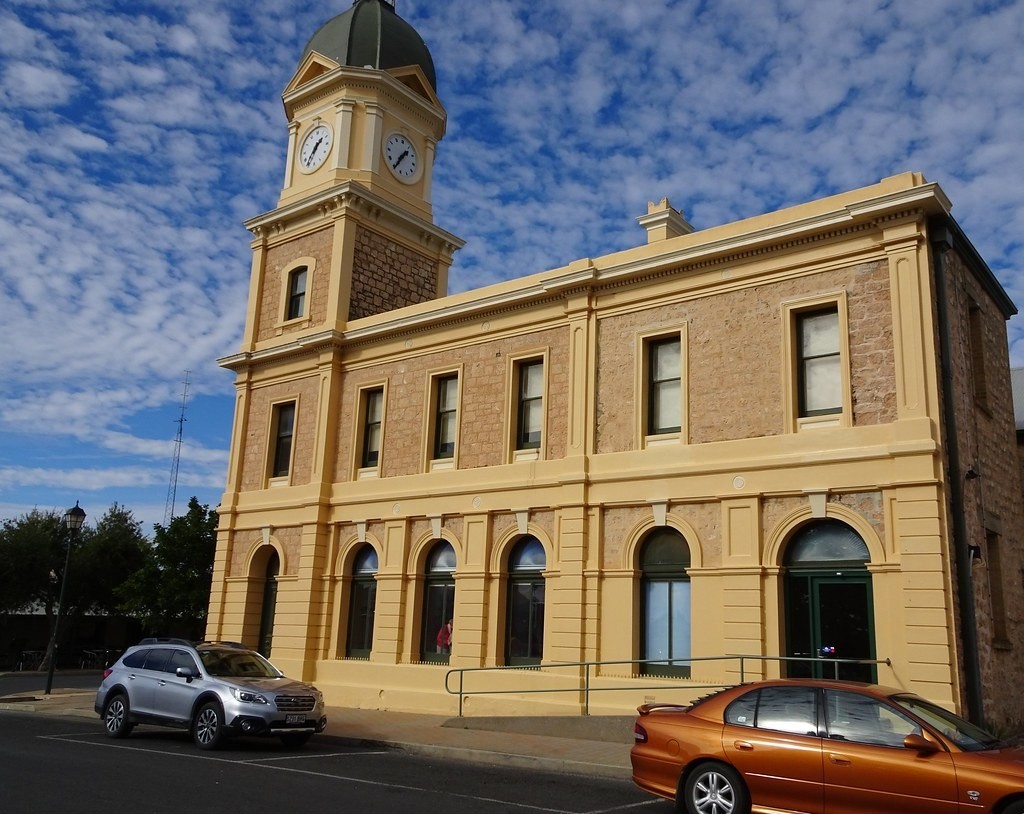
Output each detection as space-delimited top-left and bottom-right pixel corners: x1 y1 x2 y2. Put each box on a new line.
435 620 454 655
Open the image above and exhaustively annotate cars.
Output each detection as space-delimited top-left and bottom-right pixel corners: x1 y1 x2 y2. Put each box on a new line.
631 679 1024 814
94 638 327 749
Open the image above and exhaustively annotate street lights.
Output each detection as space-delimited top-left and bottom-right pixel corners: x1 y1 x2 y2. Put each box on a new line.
44 500 86 694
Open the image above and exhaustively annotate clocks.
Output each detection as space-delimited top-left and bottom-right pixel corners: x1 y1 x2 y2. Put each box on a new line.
381 128 424 185
295 121 334 175
